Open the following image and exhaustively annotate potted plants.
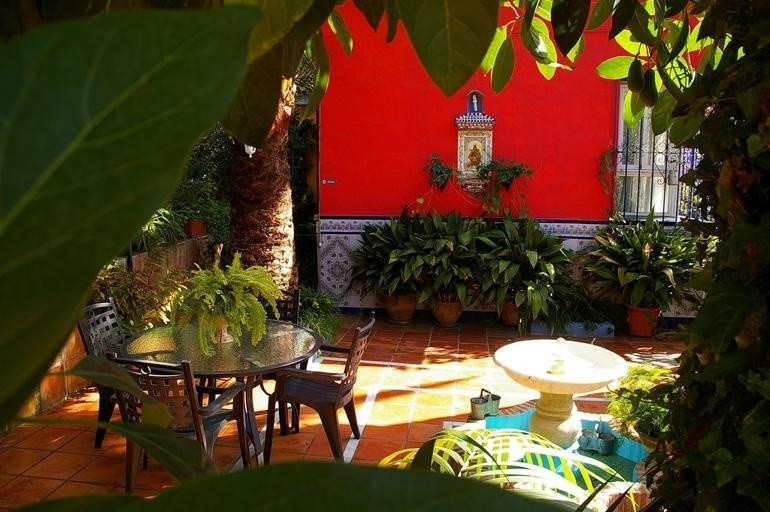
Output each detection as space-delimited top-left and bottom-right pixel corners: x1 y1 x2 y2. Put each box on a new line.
169 174 232 268
630 398 678 456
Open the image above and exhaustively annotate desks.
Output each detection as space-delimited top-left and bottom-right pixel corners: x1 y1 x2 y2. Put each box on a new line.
493 339 629 449
